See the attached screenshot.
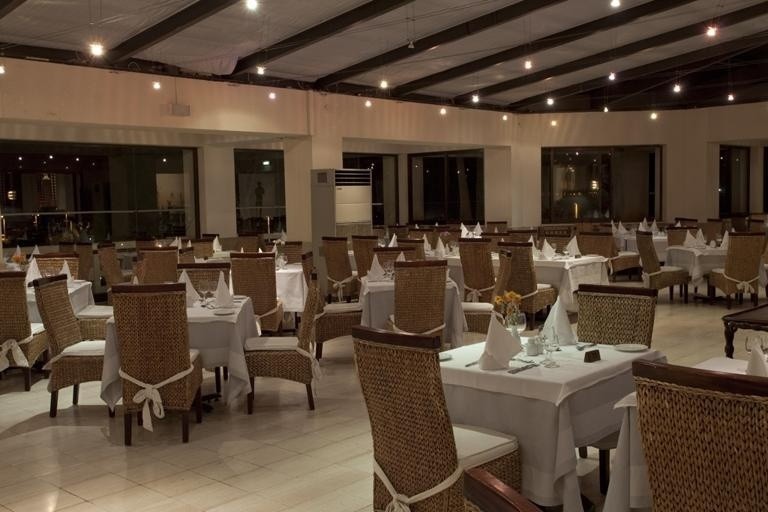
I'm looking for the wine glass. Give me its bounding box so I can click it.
[562,247,570,260]
[537,324,561,368]
[508,313,527,351]
[551,242,557,250]
[40,267,61,278]
[449,240,457,256]
[279,255,288,271]
[199,279,209,307]
[209,281,218,301]
[383,259,394,280]
